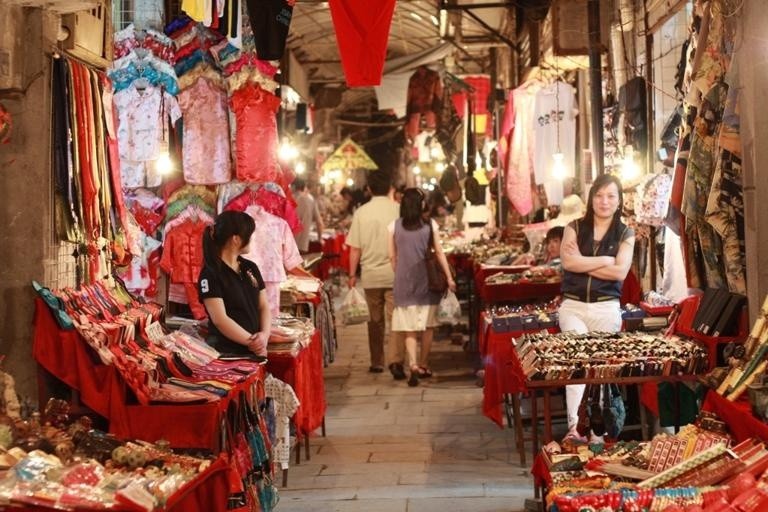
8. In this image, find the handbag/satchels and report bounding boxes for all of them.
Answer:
[604,396,626,437]
[576,402,591,437]
[221,450,249,511]
[425,251,448,292]
[257,416,275,479]
[243,426,280,512]
[227,432,262,512]
[592,399,606,437]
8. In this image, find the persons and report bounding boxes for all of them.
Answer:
[345,169,405,382]
[558,172,638,451]
[543,225,565,267]
[287,175,324,257]
[461,177,489,231]
[199,211,273,366]
[339,184,367,212]
[385,187,458,388]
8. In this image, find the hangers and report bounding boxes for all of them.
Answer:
[187,180,267,224]
[125,13,260,99]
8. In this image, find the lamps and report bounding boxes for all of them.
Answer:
[549,46,569,183]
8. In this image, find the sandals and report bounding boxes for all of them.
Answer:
[407,365,418,387]
[419,366,432,379]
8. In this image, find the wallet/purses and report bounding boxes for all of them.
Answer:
[514,330,708,381]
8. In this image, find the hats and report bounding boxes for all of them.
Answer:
[439,166,462,202]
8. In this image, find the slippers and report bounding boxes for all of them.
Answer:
[115,330,221,405]
[73,296,170,365]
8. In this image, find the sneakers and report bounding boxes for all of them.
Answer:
[369,366,383,372]
[150,355,268,404]
[32,276,145,329]
[389,363,406,380]
